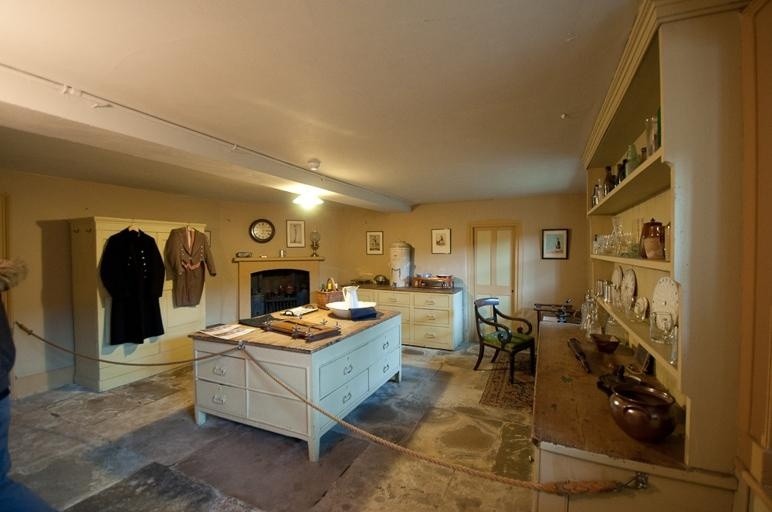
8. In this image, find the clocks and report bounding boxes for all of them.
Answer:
[249,219,275,243]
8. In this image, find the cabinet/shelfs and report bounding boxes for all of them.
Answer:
[64,215,208,394]
[412,287,463,352]
[341,285,380,312]
[529,438,735,512]
[380,286,412,346]
[588,0,757,467]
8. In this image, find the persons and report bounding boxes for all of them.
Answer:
[1,254,60,511]
[554,237,561,250]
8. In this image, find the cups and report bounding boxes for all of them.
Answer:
[651,311,675,345]
[594,231,637,258]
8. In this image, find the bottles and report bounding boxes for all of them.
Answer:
[578,287,602,340]
[591,143,641,210]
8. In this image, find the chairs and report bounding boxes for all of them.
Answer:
[473,298,535,383]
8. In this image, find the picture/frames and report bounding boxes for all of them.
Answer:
[430,228,452,254]
[285,220,307,248]
[542,229,568,260]
[365,230,384,255]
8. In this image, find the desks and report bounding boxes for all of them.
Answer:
[542,312,581,324]
[186,303,402,463]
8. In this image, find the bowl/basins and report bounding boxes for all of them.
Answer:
[324,301,377,319]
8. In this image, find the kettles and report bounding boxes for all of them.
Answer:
[326,278,337,292]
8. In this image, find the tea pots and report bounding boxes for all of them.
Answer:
[640,217,670,259]
[341,286,360,309]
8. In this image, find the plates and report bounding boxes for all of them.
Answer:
[651,276,679,332]
[611,265,623,289]
[620,269,637,310]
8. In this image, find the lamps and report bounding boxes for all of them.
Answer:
[291,189,325,213]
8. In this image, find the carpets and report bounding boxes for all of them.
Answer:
[478,346,538,415]
